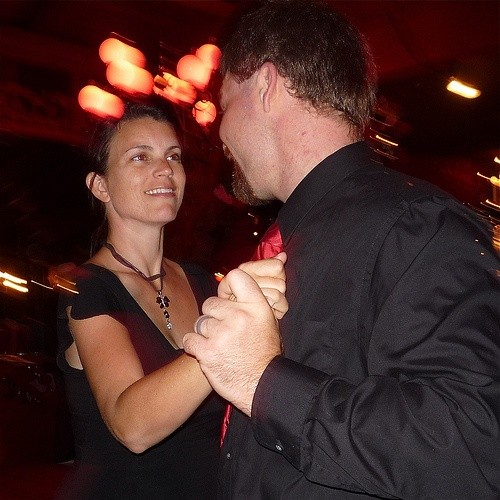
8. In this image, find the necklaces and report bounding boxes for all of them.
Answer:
[104,241,172,329]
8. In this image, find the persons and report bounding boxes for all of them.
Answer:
[216,0,500,500]
[56,96,289,500]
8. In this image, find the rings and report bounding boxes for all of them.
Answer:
[196,315,217,337]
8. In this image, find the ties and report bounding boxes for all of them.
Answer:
[219,216,283,448]
